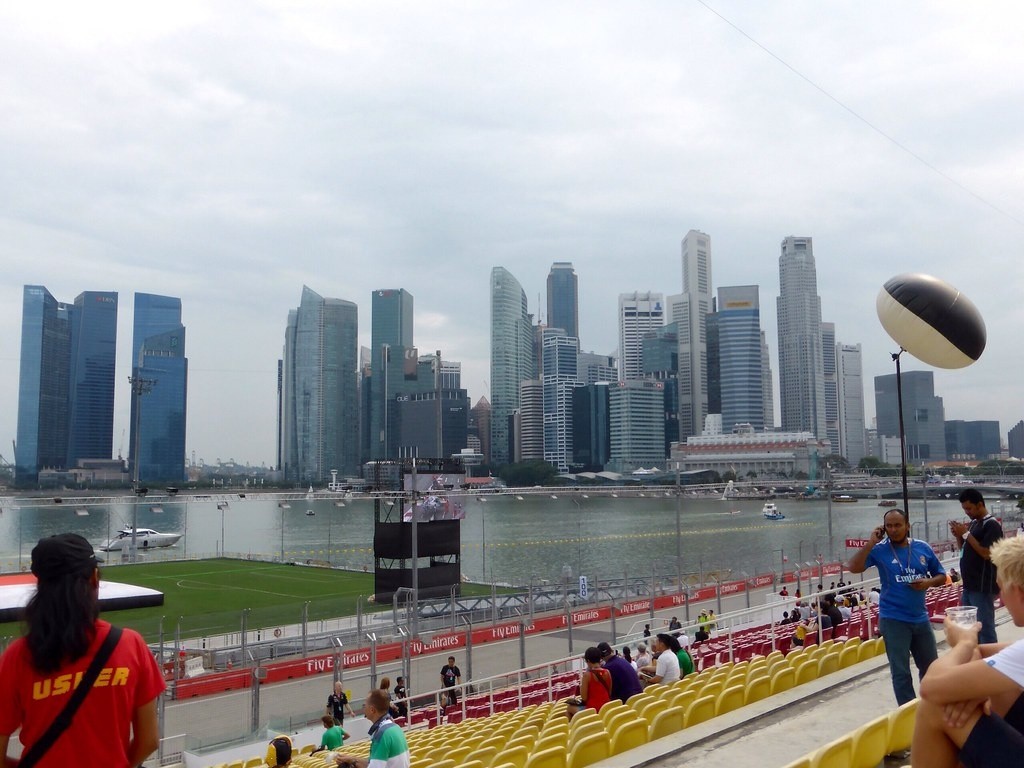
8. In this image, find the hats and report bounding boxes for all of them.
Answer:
[834,595,845,603]
[597,641,612,657]
[701,609,707,615]
[30,533,105,576]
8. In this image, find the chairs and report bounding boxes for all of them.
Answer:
[211,579,1005,768]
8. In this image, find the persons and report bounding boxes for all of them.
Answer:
[849,508,946,707]
[911,535,1024,768]
[596,642,643,705]
[707,610,716,629]
[616,632,694,690]
[394,677,408,717]
[440,656,461,704]
[380,677,400,719]
[326,682,355,728]
[567,647,612,723]
[669,617,681,638]
[950,568,960,583]
[694,627,709,644]
[697,609,710,633]
[779,578,881,649]
[336,689,410,768]
[644,624,651,645]
[320,716,351,751]
[948,488,1004,645]
[0,533,168,768]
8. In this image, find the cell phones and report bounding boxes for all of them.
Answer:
[877,528,886,537]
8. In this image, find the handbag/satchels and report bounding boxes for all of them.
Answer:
[564,697,586,706]
[440,683,448,708]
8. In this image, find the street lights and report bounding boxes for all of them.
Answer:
[127,373,159,549]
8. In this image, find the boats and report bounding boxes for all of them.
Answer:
[877,499,897,506]
[99,522,182,552]
[762,499,785,520]
[832,495,859,503]
[305,509,315,516]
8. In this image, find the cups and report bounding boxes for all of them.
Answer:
[945,605,979,627]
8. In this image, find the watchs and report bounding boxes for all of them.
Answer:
[962,531,970,540]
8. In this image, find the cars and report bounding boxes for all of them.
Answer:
[915,474,974,485]
[995,479,1024,484]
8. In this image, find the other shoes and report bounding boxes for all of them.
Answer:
[883,751,911,762]
